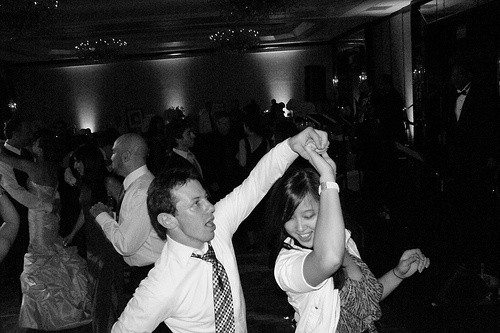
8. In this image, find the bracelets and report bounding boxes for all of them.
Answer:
[317,182,340,194]
[393,268,405,280]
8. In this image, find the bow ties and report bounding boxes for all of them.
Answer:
[456,80,475,97]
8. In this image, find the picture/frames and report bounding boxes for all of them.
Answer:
[127,109,143,130]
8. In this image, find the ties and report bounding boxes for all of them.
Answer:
[190,240,237,333]
[116,190,128,223]
[184,152,204,178]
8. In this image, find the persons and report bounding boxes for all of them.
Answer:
[110,126,330,333]
[0,188,21,262]
[0,142,94,330]
[89,133,167,333]
[0,50,500,255]
[62,143,124,333]
[274,137,431,333]
